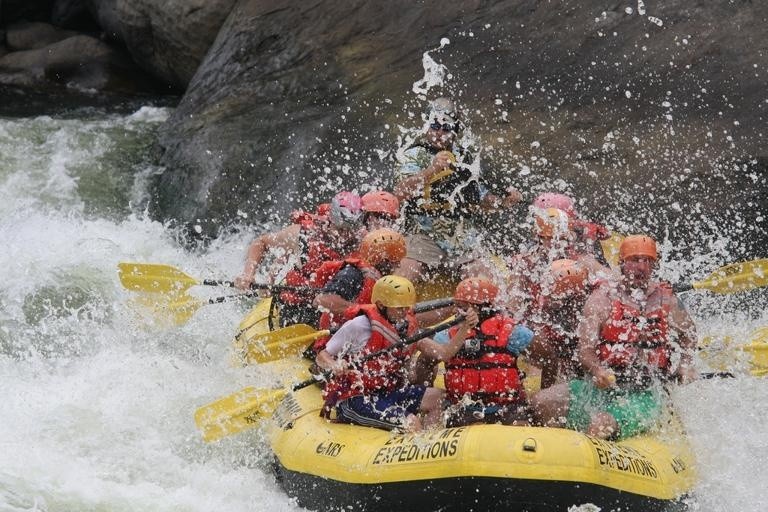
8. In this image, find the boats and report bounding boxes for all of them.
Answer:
[236,293,700,511]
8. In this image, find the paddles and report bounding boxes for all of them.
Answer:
[195,315,465,443]
[697,327,768,378]
[245,297,454,364]
[674,257,768,296]
[116,262,318,323]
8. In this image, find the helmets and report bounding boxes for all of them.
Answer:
[359,228,407,266]
[371,275,416,307]
[330,192,362,216]
[362,190,400,220]
[430,97,457,123]
[530,191,577,237]
[452,278,490,304]
[620,235,656,261]
[547,259,589,298]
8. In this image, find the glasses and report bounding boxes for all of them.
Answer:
[432,123,451,132]
